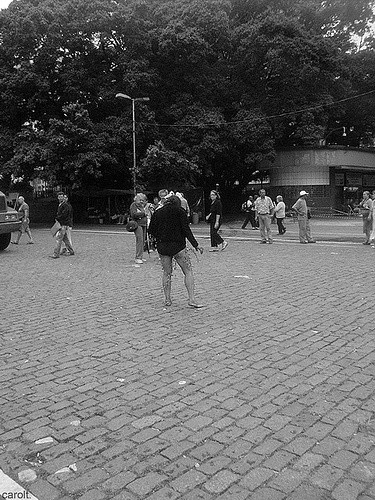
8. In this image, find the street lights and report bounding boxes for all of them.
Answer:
[116,93,150,197]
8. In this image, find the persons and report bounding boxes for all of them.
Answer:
[205,190,229,252]
[241,195,287,235]
[117,189,190,263]
[48,192,75,258]
[359,190,375,248]
[255,190,274,244]
[292,190,316,244]
[10,196,34,245]
[148,195,206,308]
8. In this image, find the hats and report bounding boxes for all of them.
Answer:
[299,191,309,196]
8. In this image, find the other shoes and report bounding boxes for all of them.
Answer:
[222,242,228,250]
[11,240,19,246]
[252,227,259,230]
[300,242,307,244]
[363,240,370,244]
[268,239,272,244]
[26,241,33,244]
[278,230,286,235]
[65,252,74,256]
[261,240,266,244]
[209,248,219,252]
[50,255,59,258]
[135,258,146,264]
[309,241,316,243]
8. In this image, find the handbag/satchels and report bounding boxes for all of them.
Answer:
[126,221,138,232]
[361,208,370,216]
